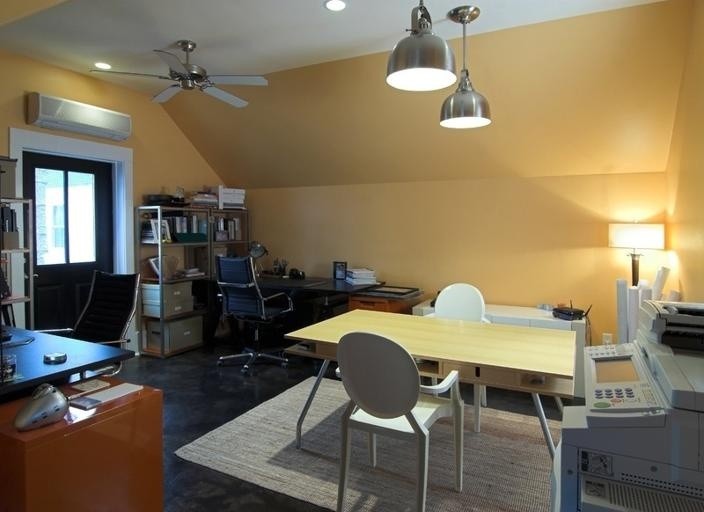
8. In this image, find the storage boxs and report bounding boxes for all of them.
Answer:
[142,296,194,318]
[147,316,203,353]
[141,281,192,301]
[0,158,17,199]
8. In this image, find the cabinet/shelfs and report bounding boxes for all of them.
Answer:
[412,299,587,412]
[349,285,421,313]
[0,199,34,329]
[135,206,211,359]
[211,209,249,337]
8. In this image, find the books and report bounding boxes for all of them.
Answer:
[61,379,111,401]
[141,185,246,278]
[345,267,376,285]
[69,382,144,410]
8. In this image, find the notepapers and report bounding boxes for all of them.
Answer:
[73,379,111,393]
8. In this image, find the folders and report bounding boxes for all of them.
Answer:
[2,207,12,231]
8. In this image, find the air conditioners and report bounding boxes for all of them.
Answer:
[26,91,132,141]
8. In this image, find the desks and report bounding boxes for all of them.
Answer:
[252,273,386,375]
[0,326,165,511]
[284,309,576,460]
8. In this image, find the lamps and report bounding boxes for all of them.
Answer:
[608,223,664,286]
[440,6,492,129]
[385,0,458,92]
[249,241,266,277]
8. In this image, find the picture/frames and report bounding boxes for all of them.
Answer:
[334,261,347,280]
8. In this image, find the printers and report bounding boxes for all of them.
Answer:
[559,299,703,511]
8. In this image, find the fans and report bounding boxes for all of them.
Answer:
[90,41,269,108]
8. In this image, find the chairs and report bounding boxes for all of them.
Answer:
[33,270,140,378]
[335,331,465,512]
[423,283,492,433]
[215,256,294,374]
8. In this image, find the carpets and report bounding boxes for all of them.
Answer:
[174,376,563,512]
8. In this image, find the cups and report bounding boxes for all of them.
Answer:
[0,355,16,382]
